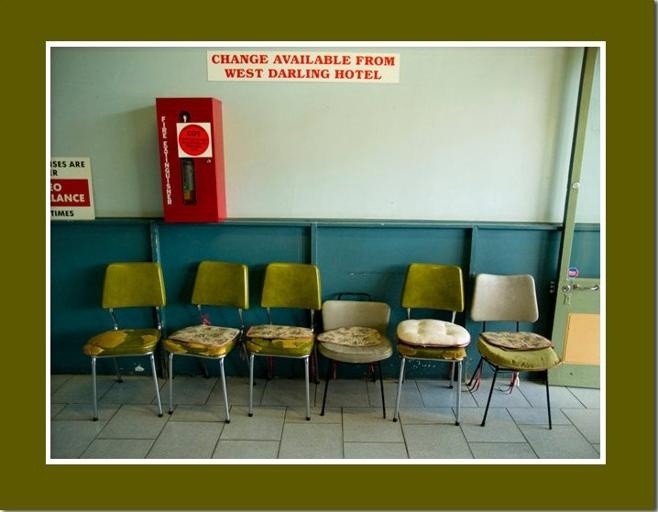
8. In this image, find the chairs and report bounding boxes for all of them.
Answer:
[82,260,562,430]
